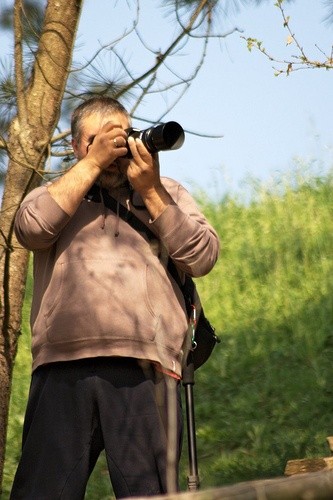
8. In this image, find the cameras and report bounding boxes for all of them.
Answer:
[116,120,185,159]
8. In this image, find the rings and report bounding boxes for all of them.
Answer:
[113,138,117,147]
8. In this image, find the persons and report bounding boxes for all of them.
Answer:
[9,97,220,500]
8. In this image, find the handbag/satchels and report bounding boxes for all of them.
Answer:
[184,274,218,371]
